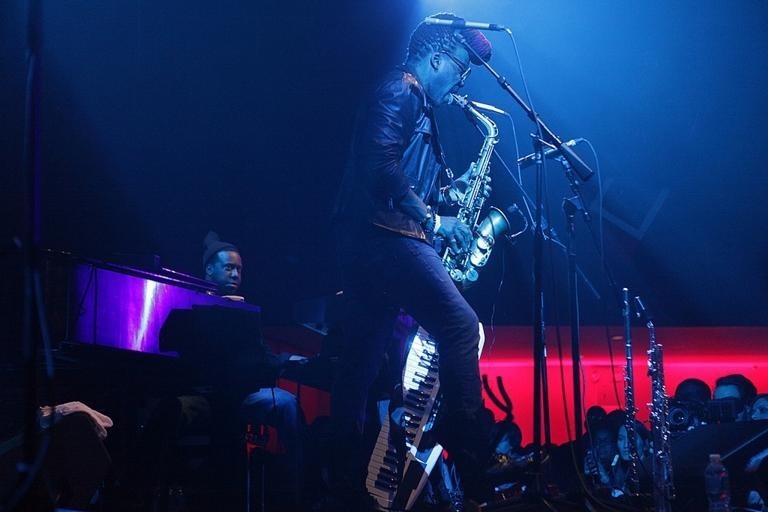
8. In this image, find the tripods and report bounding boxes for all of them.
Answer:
[456,34,594,512]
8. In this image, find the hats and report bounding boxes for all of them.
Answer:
[203,242,235,266]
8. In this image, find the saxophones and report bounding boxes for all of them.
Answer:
[622,288,639,511]
[635,294,678,511]
[441,92,511,292]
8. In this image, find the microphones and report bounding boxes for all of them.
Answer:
[424,17,499,31]
[516,139,576,169]
[470,101,505,114]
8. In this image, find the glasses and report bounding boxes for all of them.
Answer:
[441,49,472,81]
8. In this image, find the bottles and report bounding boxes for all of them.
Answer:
[703,455,730,508]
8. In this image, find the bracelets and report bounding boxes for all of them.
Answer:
[441,184,454,208]
[419,206,441,234]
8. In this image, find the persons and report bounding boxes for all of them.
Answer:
[450,374,767,510]
[328,13,492,510]
[200,241,308,493]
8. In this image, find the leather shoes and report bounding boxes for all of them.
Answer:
[462,449,549,503]
